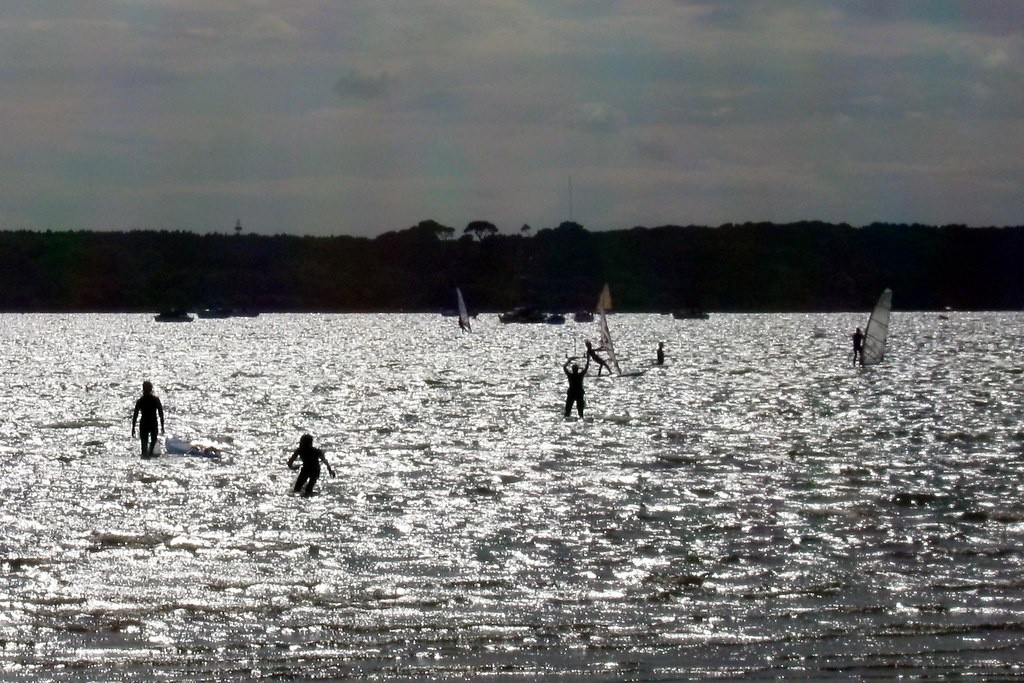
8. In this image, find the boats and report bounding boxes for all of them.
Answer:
[154,306,194,322]
[198,308,230,318]
[575,311,594,321]
[671,307,709,319]
[543,314,566,324]
[498,308,546,323]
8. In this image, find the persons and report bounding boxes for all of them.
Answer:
[132,381,165,455]
[288,434,335,497]
[657,342,664,364]
[563,357,588,418]
[853,327,866,366]
[585,341,612,376]
